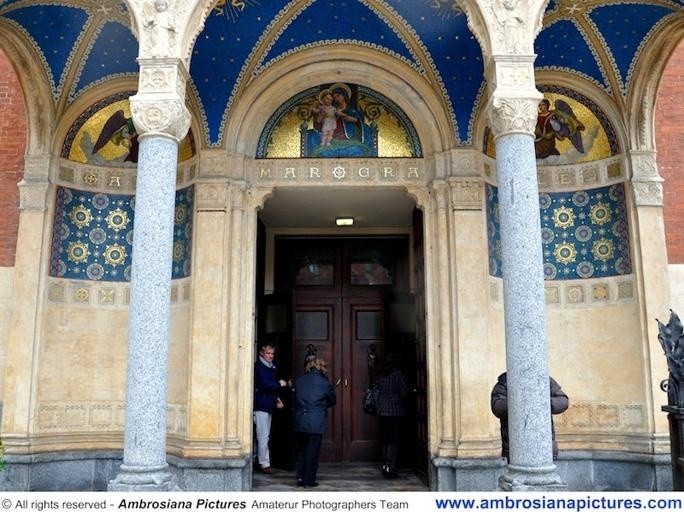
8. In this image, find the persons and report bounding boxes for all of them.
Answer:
[534,99,571,159]
[363,351,409,481]
[491,372,568,465]
[308,83,363,148]
[291,343,337,488]
[123,118,138,164]
[253,344,287,474]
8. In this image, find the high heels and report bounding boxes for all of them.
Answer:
[382,464,402,480]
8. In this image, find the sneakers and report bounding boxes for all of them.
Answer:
[260,468,273,474]
[297,477,318,488]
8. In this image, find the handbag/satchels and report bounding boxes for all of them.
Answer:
[362,383,380,416]
